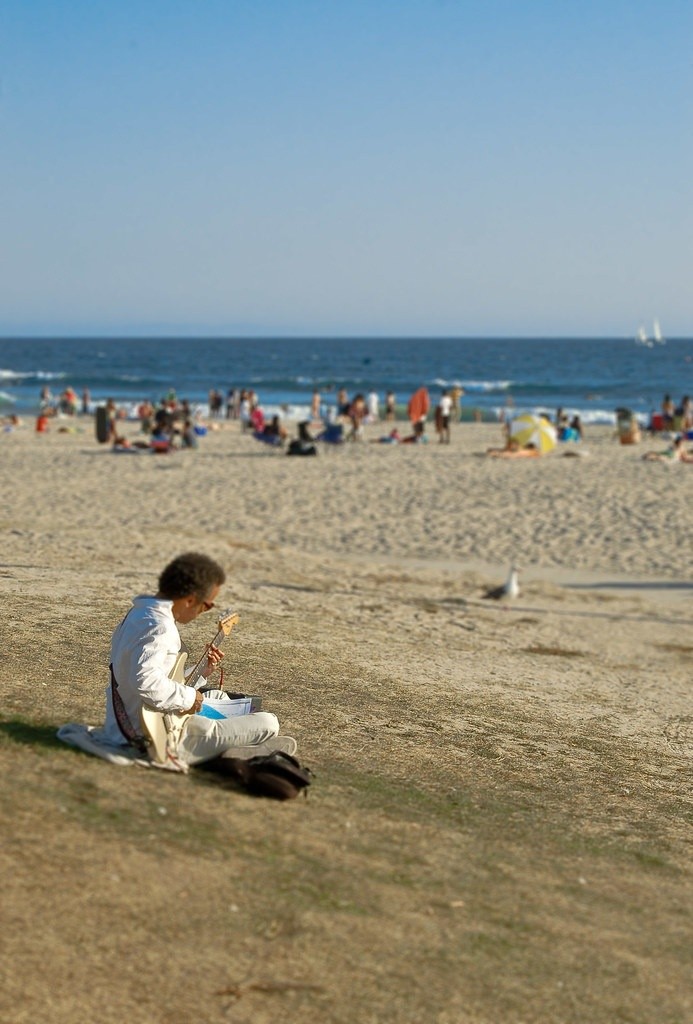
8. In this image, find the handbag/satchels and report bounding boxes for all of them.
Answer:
[236,749,316,801]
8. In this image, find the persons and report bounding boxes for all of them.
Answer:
[0,385,693,465]
[436,389,452,443]
[109,553,296,772]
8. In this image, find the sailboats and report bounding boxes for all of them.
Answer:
[633,325,653,349]
[651,318,666,345]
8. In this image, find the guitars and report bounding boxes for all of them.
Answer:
[138,607,241,764]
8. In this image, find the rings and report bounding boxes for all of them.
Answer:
[211,663,215,666]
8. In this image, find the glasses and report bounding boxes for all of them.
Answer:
[195,595,214,612]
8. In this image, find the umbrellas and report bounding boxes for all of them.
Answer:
[510,411,557,455]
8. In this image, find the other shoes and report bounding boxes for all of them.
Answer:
[221,735,297,763]
